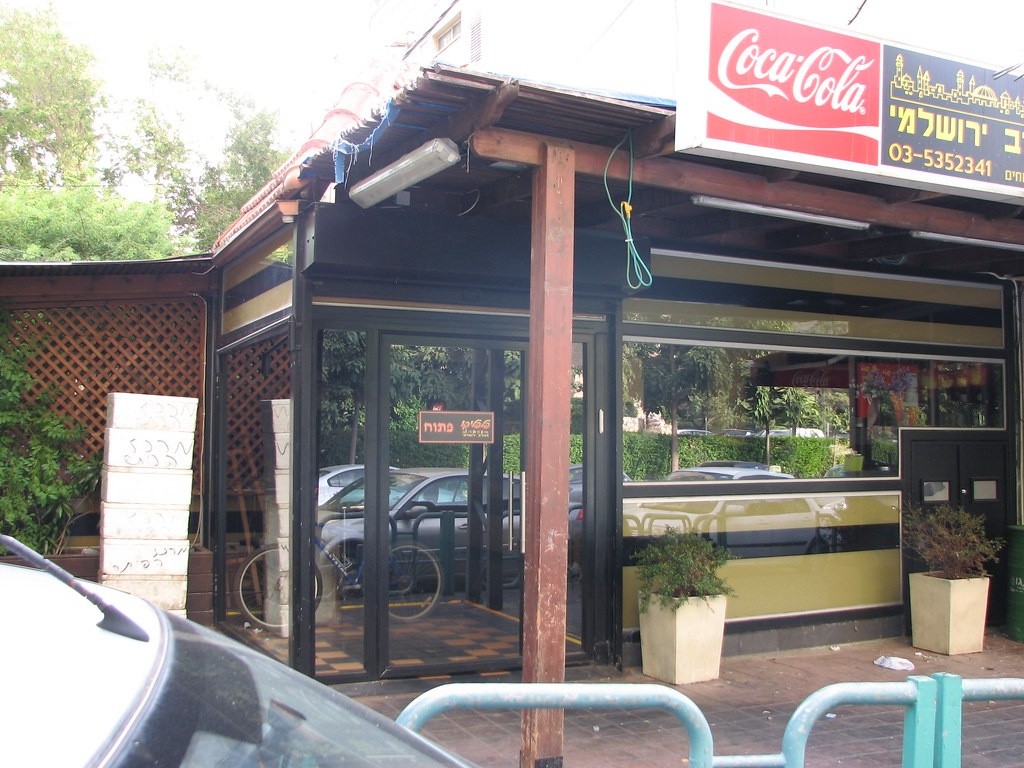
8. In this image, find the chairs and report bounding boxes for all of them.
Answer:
[424,484,439,504]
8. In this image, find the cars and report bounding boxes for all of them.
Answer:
[674,429,714,438]
[700,461,770,471]
[821,461,893,479]
[1,530,482,768]
[663,468,796,484]
[315,461,397,510]
[717,428,849,439]
[569,463,633,502]
[316,466,582,580]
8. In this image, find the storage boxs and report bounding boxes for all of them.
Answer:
[99,393,200,619]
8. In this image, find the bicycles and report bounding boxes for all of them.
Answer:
[232,514,443,631]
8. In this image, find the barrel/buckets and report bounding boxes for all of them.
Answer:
[1005,526,1024,642]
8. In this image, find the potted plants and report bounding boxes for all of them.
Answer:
[896,493,1005,655]
[0,322,216,628]
[627,524,736,684]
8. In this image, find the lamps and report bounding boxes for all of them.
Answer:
[349,138,462,209]
